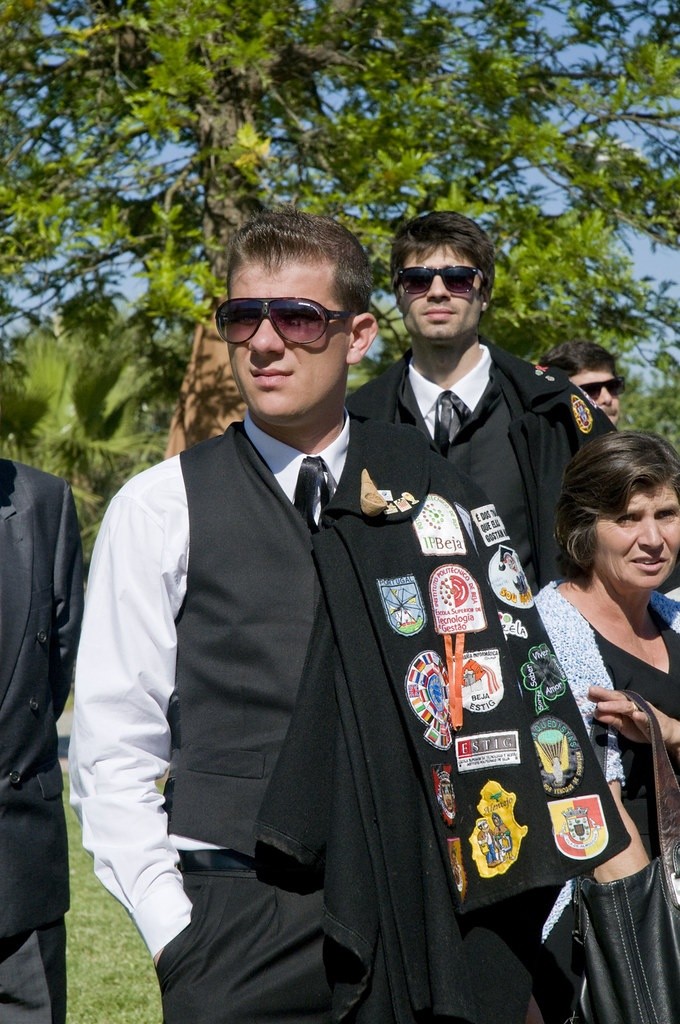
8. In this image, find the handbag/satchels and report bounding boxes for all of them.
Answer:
[571,690,680,1024]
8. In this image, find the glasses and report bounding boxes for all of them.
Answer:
[395,265,484,295]
[215,295,356,345]
[578,377,624,399]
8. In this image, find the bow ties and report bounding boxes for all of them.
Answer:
[293,456,337,536]
[433,391,473,458]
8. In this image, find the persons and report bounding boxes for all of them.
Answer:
[66,216,631,1024]
[346,211,620,587]
[539,341,620,427]
[531,430,680,990]
[0,461,86,1024]
